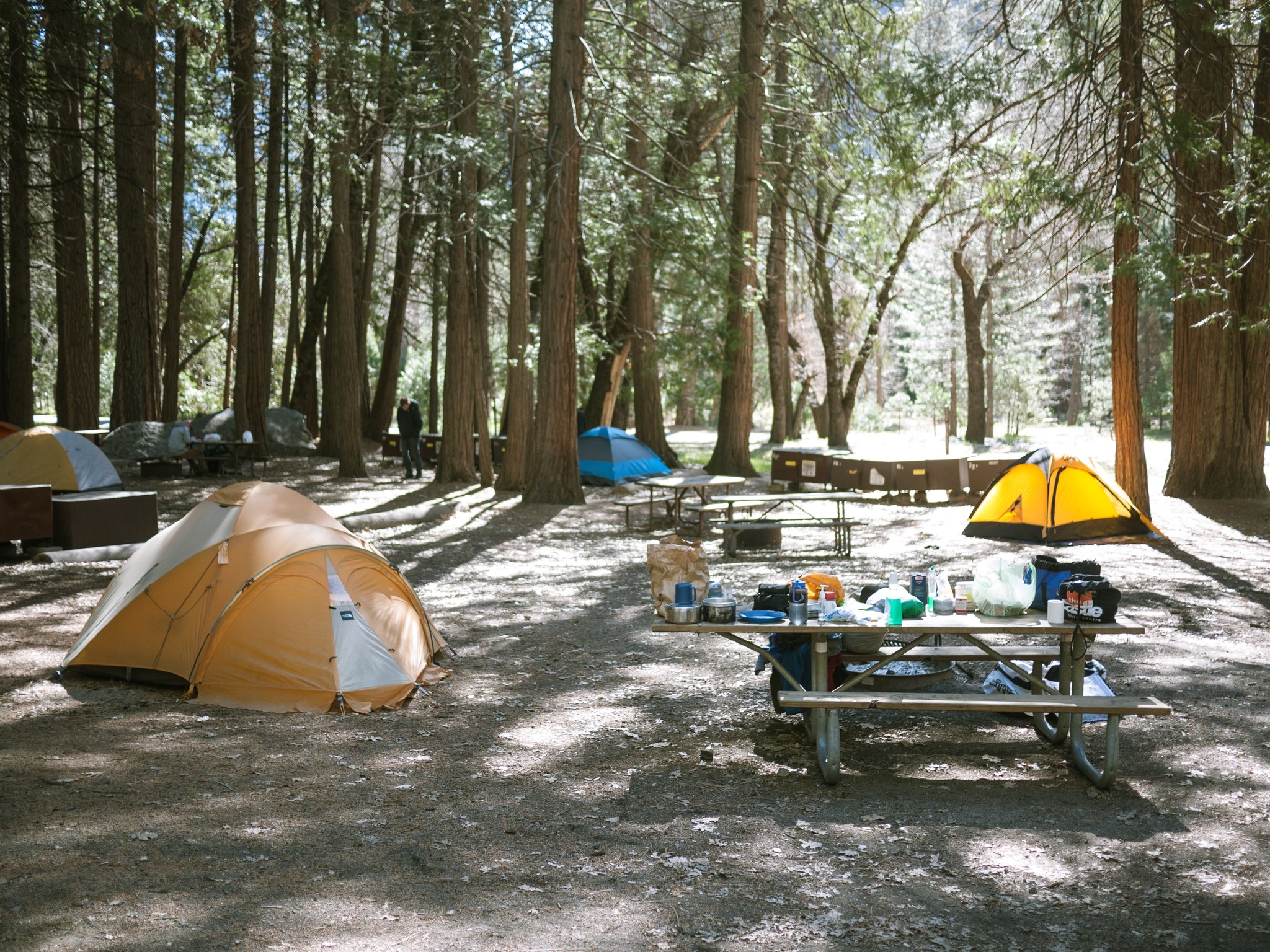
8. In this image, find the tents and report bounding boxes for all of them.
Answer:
[59,480,452,713]
[577,427,671,488]
[0,425,122,492]
[961,447,1170,546]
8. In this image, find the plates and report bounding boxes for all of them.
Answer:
[787,606,819,618]
[737,610,786,622]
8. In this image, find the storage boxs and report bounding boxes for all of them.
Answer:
[382,432,507,465]
[772,449,1027,491]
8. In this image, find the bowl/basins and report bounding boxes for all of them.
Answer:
[807,599,819,611]
[703,597,736,623]
[664,601,702,624]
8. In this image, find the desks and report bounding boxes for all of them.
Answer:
[626,474,745,537]
[711,491,862,556]
[652,607,1144,789]
[183,440,267,477]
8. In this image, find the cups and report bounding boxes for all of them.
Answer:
[825,599,837,616]
[675,583,697,604]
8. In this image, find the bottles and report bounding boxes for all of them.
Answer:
[789,580,808,624]
[927,566,936,611]
[954,581,974,600]
[885,572,903,625]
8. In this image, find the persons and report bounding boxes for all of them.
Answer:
[396,397,424,479]
[167,421,210,476]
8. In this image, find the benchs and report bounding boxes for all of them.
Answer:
[614,493,868,557]
[760,645,1171,787]
[172,454,273,477]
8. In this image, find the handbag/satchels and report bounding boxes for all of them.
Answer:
[981,658,1123,722]
[971,554,1037,617]
[1055,574,1122,624]
[787,573,845,606]
[865,583,925,617]
[753,583,791,615]
[1031,555,1101,610]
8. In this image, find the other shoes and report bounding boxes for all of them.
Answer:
[415,472,422,479]
[403,474,413,478]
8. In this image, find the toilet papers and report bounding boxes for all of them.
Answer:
[1047,599,1065,623]
